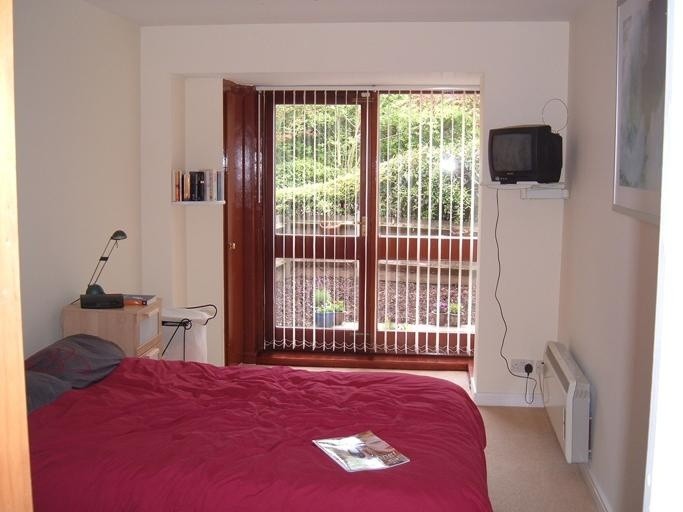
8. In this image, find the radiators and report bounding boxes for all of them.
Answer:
[542,340,590,464]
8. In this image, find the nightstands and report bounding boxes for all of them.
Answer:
[61,294,162,358]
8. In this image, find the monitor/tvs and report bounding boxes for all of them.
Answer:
[488,124,563,184]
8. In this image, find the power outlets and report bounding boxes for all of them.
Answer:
[509,359,536,375]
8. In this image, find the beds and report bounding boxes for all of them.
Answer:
[24,356,491,512]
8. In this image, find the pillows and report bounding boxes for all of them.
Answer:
[25,335,125,417]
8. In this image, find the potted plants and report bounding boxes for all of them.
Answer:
[312,289,344,327]
[448,304,459,326]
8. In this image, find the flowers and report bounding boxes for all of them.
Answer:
[434,301,446,312]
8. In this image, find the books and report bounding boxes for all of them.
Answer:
[171,168,224,202]
[122,293,158,306]
[310,429,411,473]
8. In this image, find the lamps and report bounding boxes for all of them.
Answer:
[79,229,127,308]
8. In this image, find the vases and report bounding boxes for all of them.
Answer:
[432,309,446,325]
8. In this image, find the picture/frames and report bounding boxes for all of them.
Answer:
[610,2,661,224]
